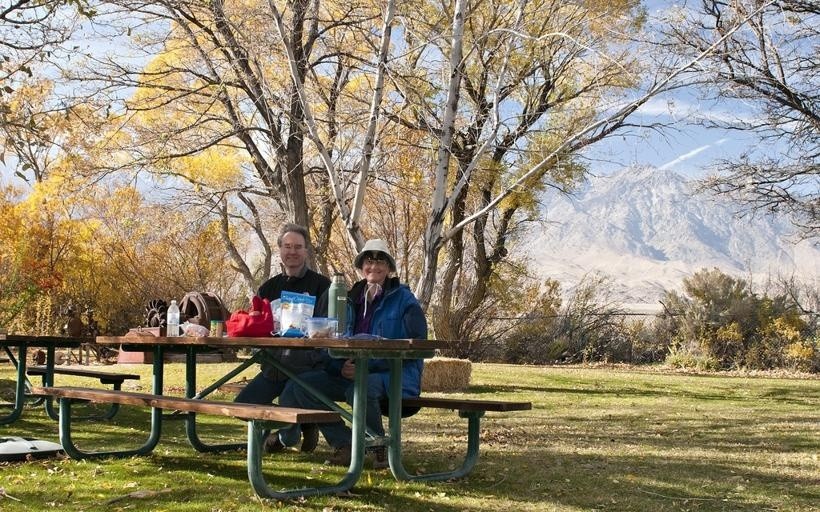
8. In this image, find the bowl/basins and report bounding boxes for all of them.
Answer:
[305,316,339,339]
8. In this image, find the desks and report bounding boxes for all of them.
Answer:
[0,335,86,421]
[95,334,452,497]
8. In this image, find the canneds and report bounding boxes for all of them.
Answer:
[210,320,224,337]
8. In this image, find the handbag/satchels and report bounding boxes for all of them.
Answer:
[226,296,273,337]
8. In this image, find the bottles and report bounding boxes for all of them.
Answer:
[166,299,180,337]
[327,271,349,339]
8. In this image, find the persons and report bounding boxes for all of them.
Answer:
[294,238,427,470]
[232,223,333,452]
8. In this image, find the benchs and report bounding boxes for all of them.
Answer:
[28,363,140,421]
[220,378,532,481]
[27,385,353,500]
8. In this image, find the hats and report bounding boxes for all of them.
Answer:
[354,240,396,272]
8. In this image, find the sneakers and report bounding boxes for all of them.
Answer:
[264,431,284,453]
[301,423,318,452]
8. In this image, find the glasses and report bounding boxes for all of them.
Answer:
[365,258,386,265]
